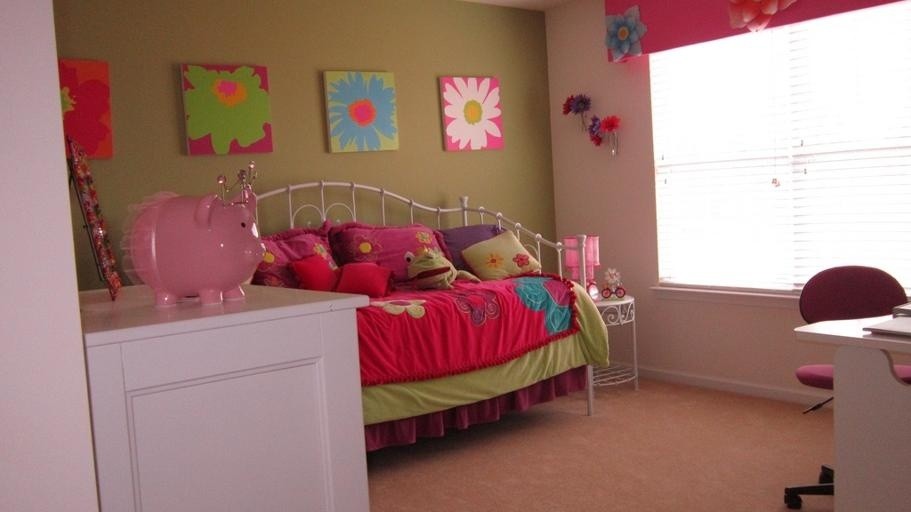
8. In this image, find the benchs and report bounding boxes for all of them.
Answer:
[255,180,593,451]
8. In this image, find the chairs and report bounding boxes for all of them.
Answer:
[783,266,911,510]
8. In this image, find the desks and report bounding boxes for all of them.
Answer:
[793,315,911,512]
[587,294,639,391]
[79,285,370,512]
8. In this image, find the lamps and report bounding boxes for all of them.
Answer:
[562,235,600,284]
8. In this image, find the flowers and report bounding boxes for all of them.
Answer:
[562,93,622,159]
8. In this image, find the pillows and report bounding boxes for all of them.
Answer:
[257,219,542,298]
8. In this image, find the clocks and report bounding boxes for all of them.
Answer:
[587,283,600,302]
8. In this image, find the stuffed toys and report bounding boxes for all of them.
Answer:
[407,248,482,291]
